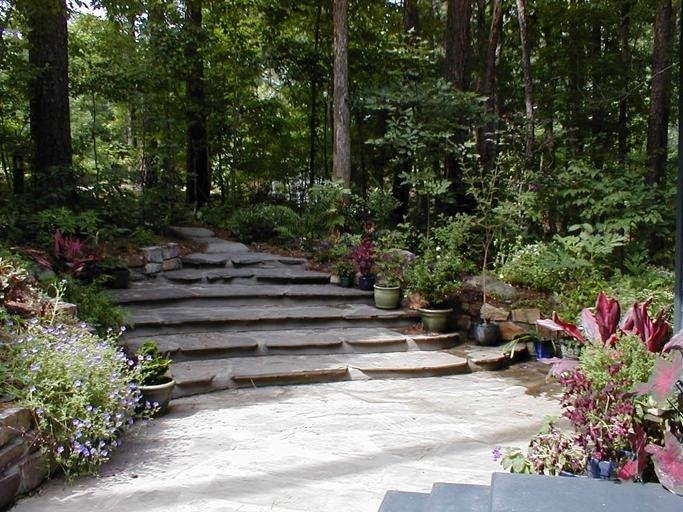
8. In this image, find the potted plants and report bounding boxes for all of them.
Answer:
[335,233,462,331]
[100,252,129,289]
[448,139,532,346]
[124,340,176,419]
